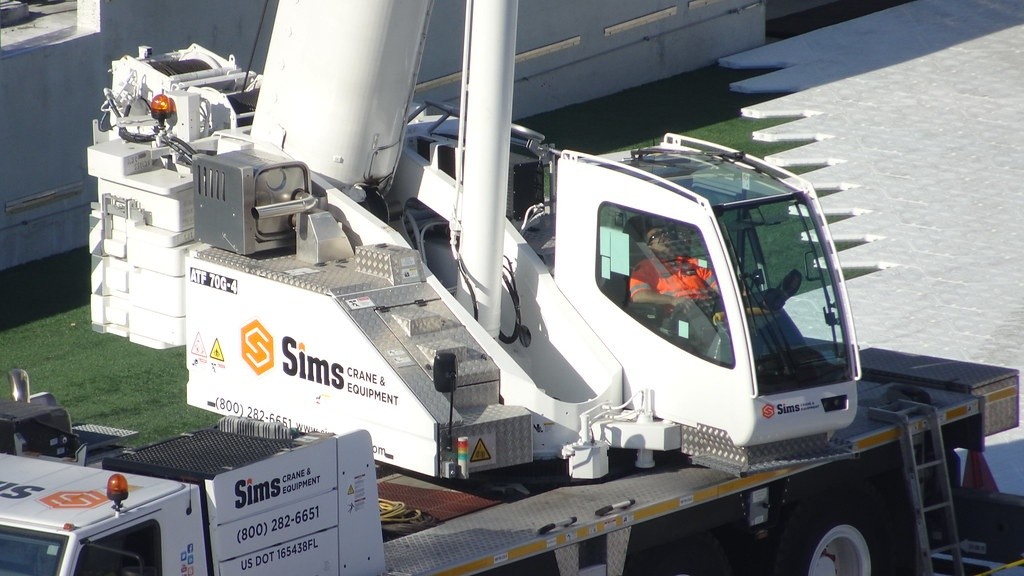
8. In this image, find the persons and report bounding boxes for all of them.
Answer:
[630,226,802,354]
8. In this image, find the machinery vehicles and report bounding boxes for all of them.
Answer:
[80,0,868,484]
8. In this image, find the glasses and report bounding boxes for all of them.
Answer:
[647,229,668,246]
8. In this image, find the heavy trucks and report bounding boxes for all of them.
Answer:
[0,337,1021,576]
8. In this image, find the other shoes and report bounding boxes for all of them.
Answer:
[770,269,803,309]
